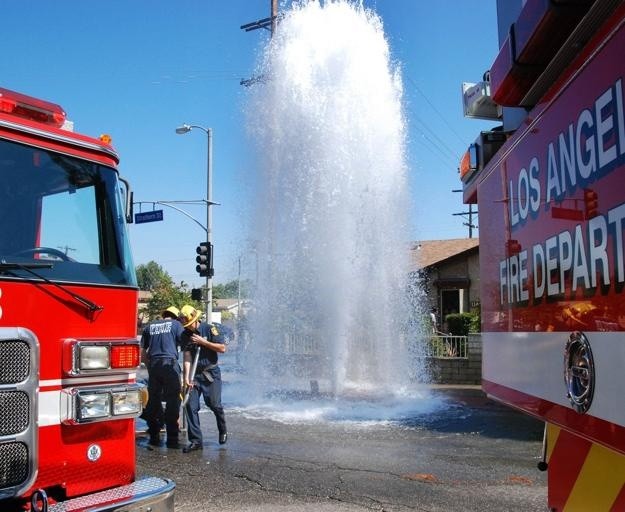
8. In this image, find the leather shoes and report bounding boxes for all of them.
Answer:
[219,433,227,444]
[183,442,203,453]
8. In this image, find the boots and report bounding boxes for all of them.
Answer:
[148,429,163,446]
[167,433,182,448]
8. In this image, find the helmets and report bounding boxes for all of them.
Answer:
[179,305,201,327]
[163,306,180,318]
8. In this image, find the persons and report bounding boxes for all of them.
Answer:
[430,305,443,336]
[136,305,190,448]
[177,304,229,453]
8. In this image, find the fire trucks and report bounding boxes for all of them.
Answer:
[0,82,179,511]
[455,1,625,512]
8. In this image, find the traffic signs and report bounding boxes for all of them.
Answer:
[134,209,164,225]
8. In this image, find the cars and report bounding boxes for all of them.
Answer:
[210,321,235,345]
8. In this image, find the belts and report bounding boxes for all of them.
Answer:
[196,360,218,383]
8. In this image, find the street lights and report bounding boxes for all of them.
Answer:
[172,120,214,328]
[237,248,258,320]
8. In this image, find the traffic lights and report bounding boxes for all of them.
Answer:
[194,242,213,277]
[191,287,204,301]
